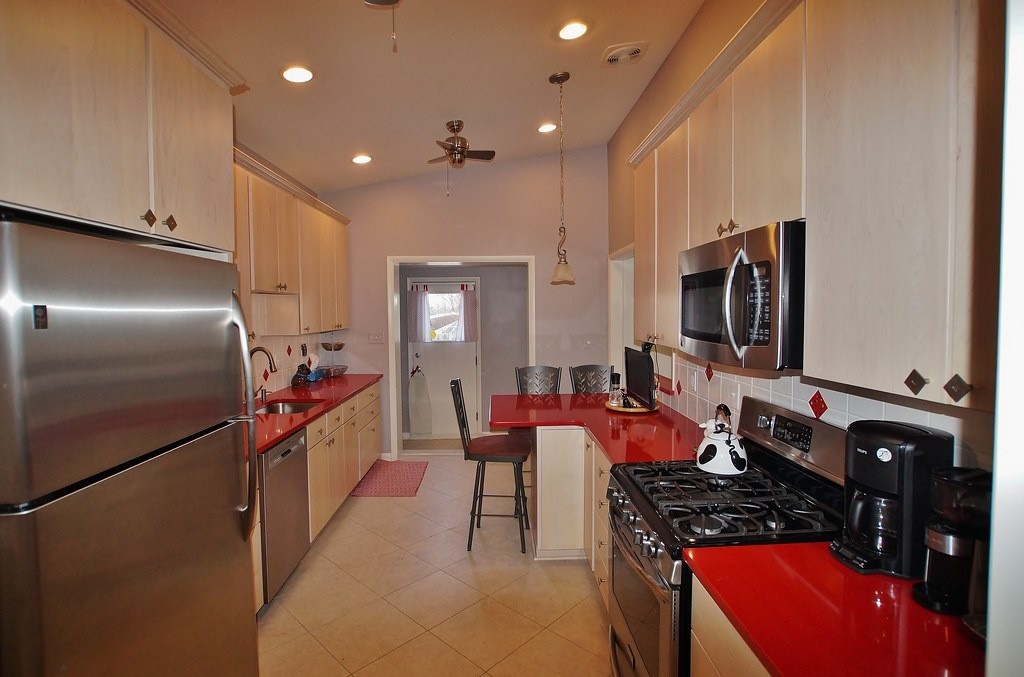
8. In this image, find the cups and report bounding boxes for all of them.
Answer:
[609,390,621,406]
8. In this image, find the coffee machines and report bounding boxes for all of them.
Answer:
[828,419,954,579]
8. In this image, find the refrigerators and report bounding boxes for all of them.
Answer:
[0,220,258,677]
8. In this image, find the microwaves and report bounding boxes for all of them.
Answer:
[678,221,805,371]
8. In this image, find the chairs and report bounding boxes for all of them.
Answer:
[569,364,615,394]
[514,366,562,394]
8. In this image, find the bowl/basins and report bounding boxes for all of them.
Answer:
[317,365,347,376]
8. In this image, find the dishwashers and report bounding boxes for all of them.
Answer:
[257,427,310,603]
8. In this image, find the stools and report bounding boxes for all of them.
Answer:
[450,379,533,553]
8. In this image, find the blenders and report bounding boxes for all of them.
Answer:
[912,466,991,615]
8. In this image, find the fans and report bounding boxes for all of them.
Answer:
[429,121,497,164]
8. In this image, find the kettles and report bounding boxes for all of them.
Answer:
[696,403,747,475]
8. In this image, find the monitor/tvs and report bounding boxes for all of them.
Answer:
[624,347,656,412]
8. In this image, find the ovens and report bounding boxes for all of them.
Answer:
[608,505,691,677]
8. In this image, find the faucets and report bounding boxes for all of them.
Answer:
[261,389,273,403]
[244,346,277,409]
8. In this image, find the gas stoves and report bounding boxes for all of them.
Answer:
[610,394,846,558]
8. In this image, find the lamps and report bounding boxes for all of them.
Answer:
[551,71,574,285]
[447,153,467,168]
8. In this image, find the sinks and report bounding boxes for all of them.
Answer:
[254,402,321,414]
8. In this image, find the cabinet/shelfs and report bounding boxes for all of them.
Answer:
[251,170,299,294]
[0,0,238,255]
[800,0,1006,413]
[322,213,347,331]
[299,200,321,334]
[583,433,609,607]
[308,381,383,542]
[690,0,806,250]
[237,164,249,336]
[632,118,693,349]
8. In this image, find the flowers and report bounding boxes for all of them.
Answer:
[690,370,696,391]
[730,381,740,411]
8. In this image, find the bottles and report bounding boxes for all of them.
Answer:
[611,373,620,390]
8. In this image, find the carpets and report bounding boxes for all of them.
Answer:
[351,458,429,497]
